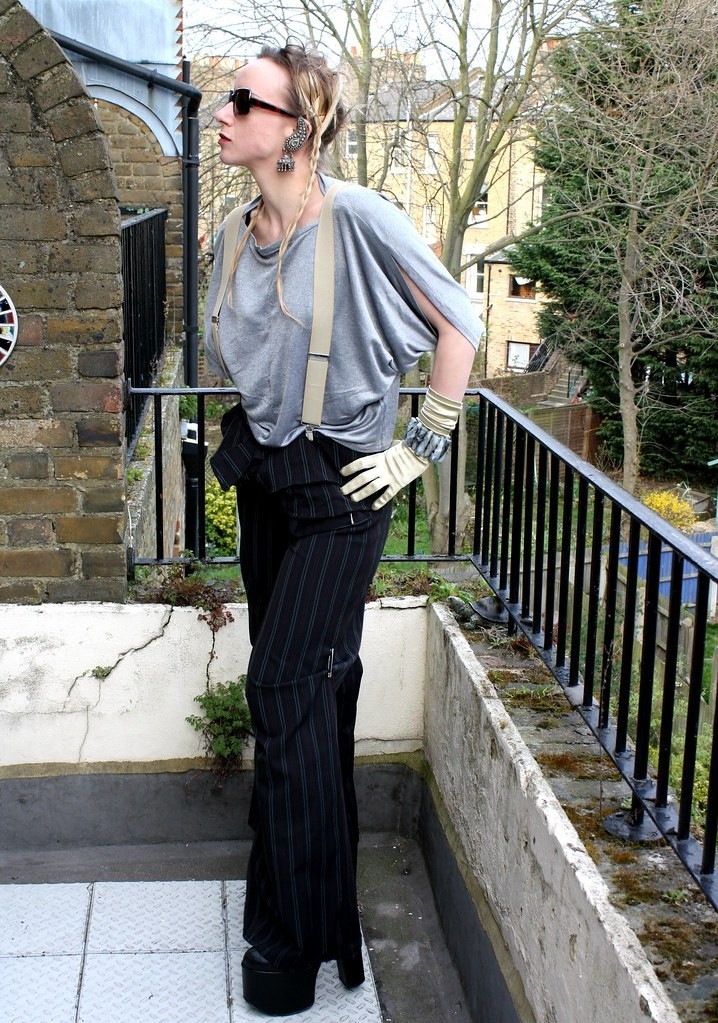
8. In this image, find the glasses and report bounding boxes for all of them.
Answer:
[228,88,315,139]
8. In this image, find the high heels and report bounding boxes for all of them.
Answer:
[241,930,366,1017]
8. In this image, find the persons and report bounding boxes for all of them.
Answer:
[203,43,481,1013]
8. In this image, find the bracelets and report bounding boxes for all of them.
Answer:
[405,416,451,464]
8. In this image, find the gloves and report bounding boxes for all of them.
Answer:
[339,384,463,510]
